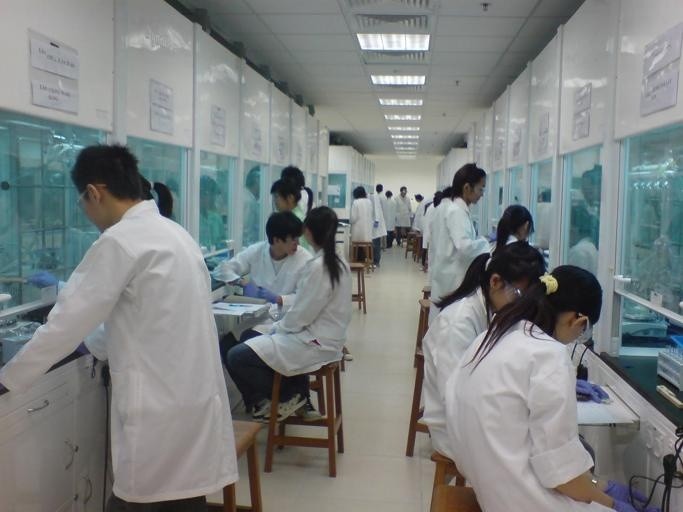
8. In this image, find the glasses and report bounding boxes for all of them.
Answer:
[501,281,525,304]
[75,183,109,206]
[577,312,594,342]
[402,190,407,193]
[470,184,487,196]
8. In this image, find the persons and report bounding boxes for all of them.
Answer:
[244,166,259,244]
[427,164,497,326]
[580,165,601,206]
[487,206,534,269]
[0,143,239,512]
[568,207,599,274]
[26,174,172,362]
[0,175,86,267]
[270,166,433,270]
[422,241,609,473]
[212,210,322,419]
[198,175,228,250]
[446,265,659,512]
[226,206,352,424]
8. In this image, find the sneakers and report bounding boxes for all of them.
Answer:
[343,347,354,362]
[251,391,323,425]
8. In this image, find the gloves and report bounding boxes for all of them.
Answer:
[27,270,60,294]
[485,229,499,243]
[576,379,610,404]
[602,479,661,512]
[373,221,378,229]
[242,282,278,305]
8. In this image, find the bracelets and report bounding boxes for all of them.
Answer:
[236,277,243,285]
[592,475,598,489]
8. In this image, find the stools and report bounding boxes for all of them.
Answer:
[348,260,368,313]
[430,453,466,486]
[400,346,431,457]
[415,298,435,365]
[426,484,482,512]
[255,355,350,476]
[204,415,264,510]
[419,284,431,299]
[352,227,425,272]
